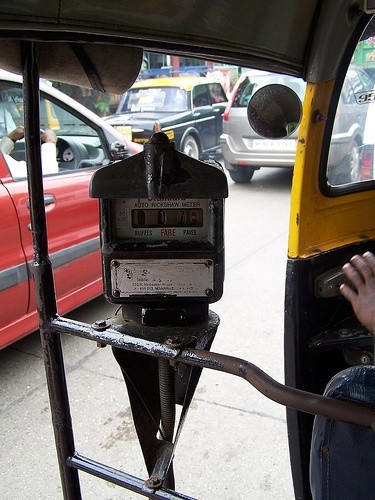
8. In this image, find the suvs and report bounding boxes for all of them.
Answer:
[98,66,228,162]
[218,64,375,185]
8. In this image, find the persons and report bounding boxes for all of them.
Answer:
[340,251,375,334]
[0,125,59,178]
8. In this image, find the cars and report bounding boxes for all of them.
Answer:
[0,66,143,354]
